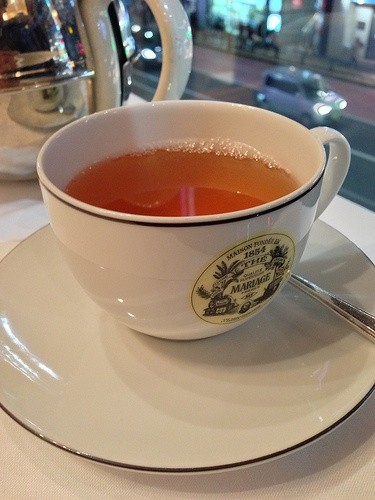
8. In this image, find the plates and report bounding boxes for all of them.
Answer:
[0,222,375,476]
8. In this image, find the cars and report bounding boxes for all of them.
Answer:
[124,22,164,73]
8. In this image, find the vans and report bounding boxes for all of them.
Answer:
[255,66,347,130]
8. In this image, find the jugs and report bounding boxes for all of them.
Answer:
[0,0,194,183]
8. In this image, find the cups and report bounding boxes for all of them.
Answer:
[36,99,351,341]
